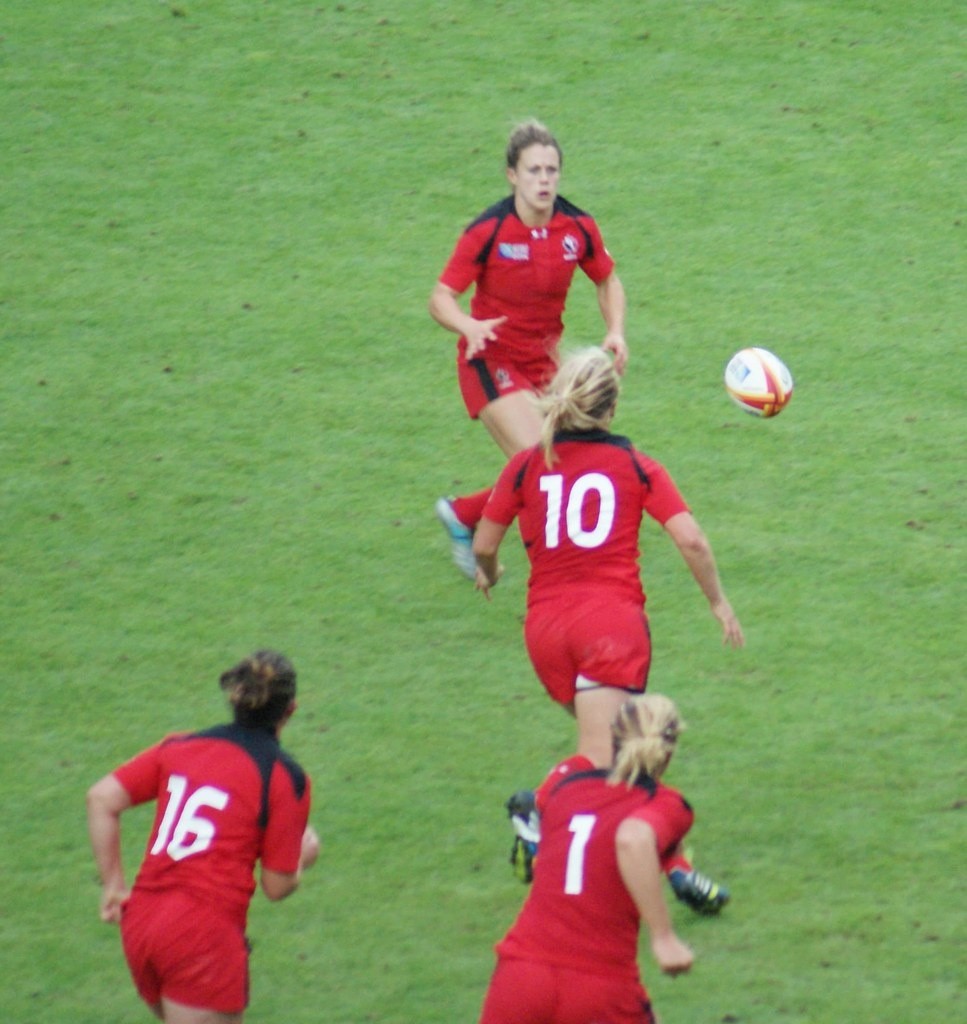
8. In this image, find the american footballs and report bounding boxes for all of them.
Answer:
[726,347,794,419]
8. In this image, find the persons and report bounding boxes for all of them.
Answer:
[428,121,630,580]
[469,348,747,1024]
[84,648,322,1024]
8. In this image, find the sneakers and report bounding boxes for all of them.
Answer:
[667,866,731,917]
[433,493,477,583]
[503,787,543,886]
